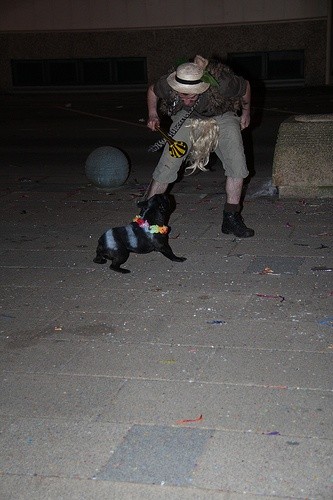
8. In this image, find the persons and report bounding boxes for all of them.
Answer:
[145,55,256,239]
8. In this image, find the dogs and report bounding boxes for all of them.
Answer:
[93,193,187,274]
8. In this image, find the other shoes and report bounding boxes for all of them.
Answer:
[222,211,254,237]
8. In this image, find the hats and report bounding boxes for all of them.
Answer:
[166,62,210,93]
